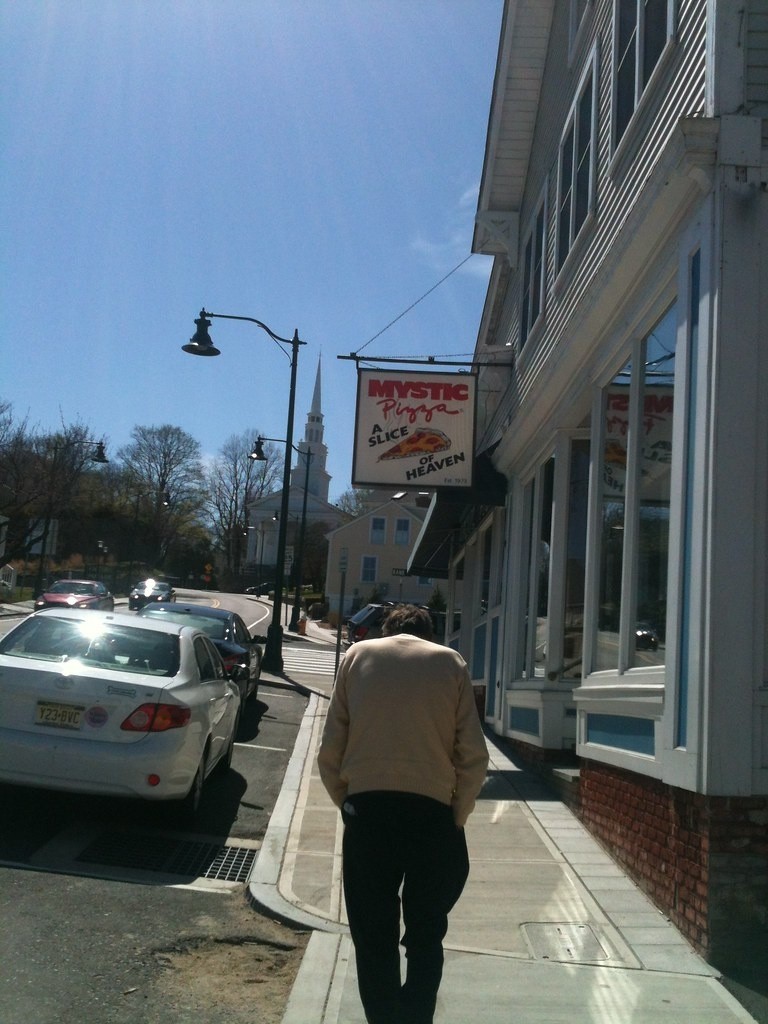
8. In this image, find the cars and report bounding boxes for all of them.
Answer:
[244,582,277,596]
[0,604,253,819]
[32,579,116,615]
[99,600,269,725]
[128,578,177,611]
[637,626,659,653]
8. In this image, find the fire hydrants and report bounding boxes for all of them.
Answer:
[296,613,308,635]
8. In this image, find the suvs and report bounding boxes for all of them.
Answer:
[342,602,461,651]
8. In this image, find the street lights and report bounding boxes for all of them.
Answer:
[97,539,104,581]
[248,526,264,598]
[246,433,316,632]
[123,489,172,597]
[102,546,109,582]
[182,306,311,675]
[32,440,110,600]
[272,508,301,592]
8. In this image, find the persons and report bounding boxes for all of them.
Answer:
[318,605,489,1024]
